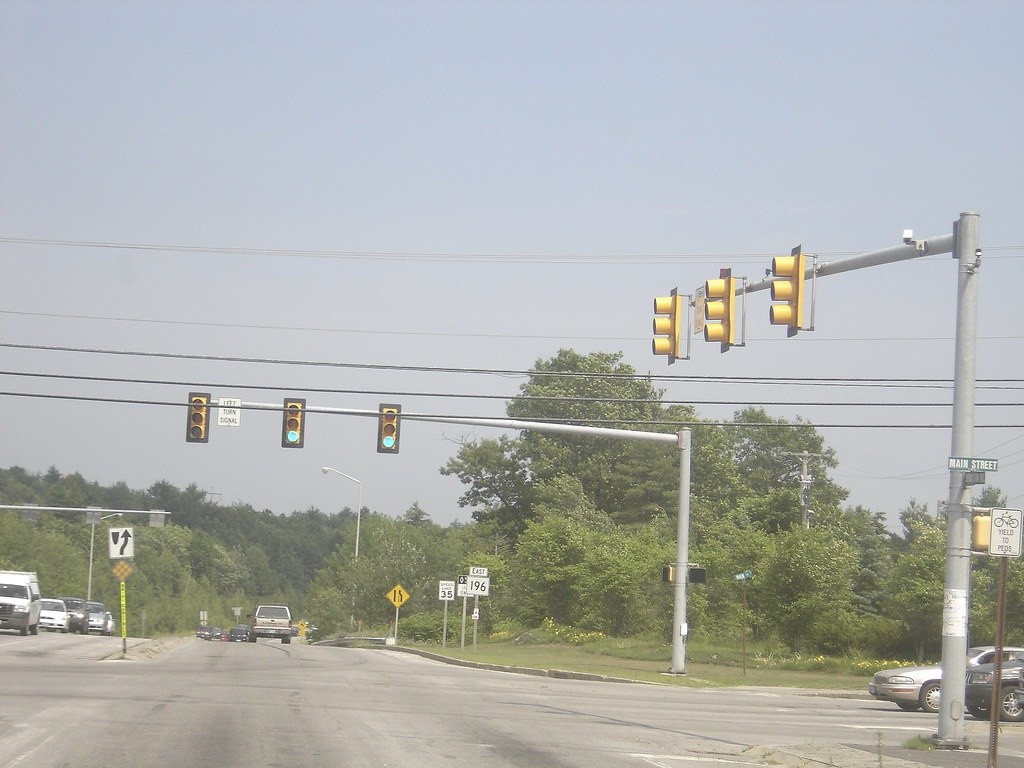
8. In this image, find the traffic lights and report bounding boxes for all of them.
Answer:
[770,244,806,339]
[704,267,734,353]
[376,403,402,454]
[282,397,306,449]
[652,286,682,365]
[186,392,211,443]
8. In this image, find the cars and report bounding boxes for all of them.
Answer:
[107,615,117,636]
[963,652,1024,723]
[195,626,249,642]
[866,645,1024,713]
[38,597,71,635]
[85,601,111,636]
[57,595,90,635]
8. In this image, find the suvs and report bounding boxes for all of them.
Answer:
[245,604,293,644]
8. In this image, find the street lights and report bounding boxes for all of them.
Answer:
[322,466,363,632]
[86,512,124,602]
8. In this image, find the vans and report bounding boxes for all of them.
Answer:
[0,569,43,637]
